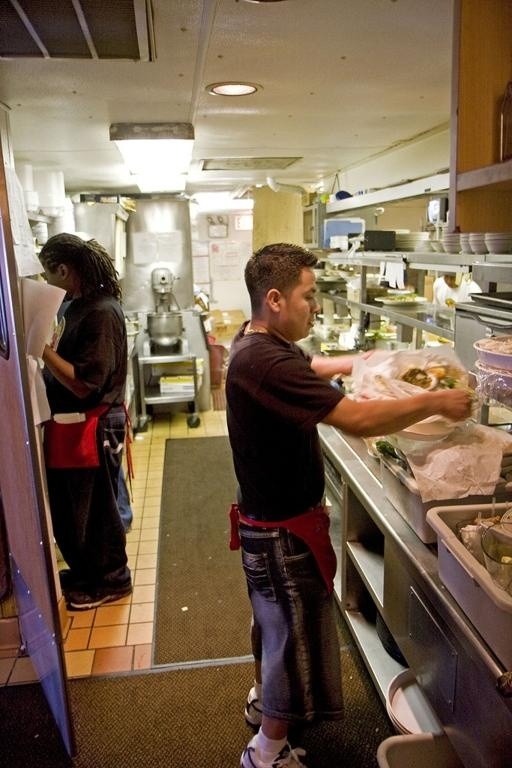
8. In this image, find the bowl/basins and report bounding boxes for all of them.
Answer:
[429,233,511,255]
[312,269,327,281]
[338,270,355,278]
[473,335,512,399]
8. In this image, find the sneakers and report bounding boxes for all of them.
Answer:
[240,735,308,767]
[244,687,266,725]
[59,570,133,610]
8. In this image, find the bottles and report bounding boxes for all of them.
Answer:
[496,82,511,164]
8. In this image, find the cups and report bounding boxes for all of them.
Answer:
[456,518,512,597]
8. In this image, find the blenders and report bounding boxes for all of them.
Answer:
[147,267,185,349]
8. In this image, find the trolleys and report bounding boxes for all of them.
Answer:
[137,336,201,431]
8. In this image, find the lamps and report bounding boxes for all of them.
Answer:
[109,122,196,194]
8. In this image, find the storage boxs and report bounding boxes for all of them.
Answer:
[372,442,512,544]
[426,501,512,673]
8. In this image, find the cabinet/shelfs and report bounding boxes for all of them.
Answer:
[316,251,486,350]
[301,204,325,250]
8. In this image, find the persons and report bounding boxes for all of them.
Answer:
[224,244,474,768]
[35,230,134,612]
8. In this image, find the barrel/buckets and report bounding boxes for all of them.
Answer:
[147,312,184,347]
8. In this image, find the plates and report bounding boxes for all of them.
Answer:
[321,274,340,280]
[374,294,427,307]
[395,231,427,250]
